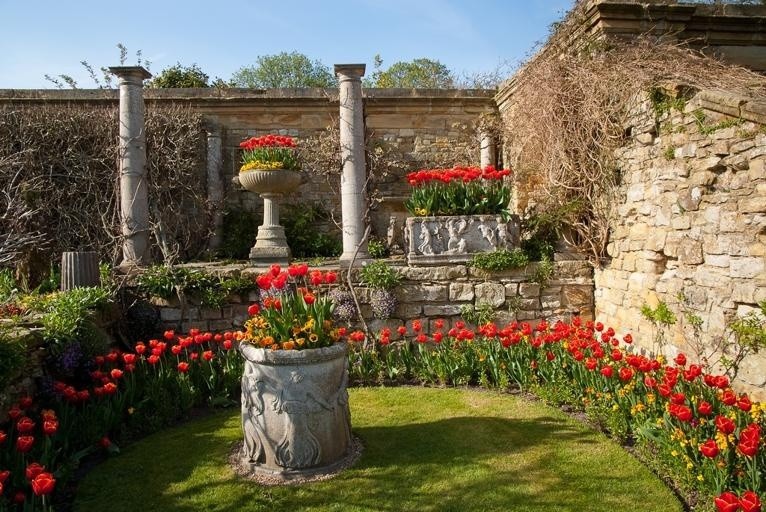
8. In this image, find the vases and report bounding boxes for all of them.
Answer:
[239,339,353,478]
[238,170,302,198]
[406,213,521,267]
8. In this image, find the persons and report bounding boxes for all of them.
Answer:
[384,216,397,250]
[418,217,514,254]
[241,362,263,463]
[275,370,334,467]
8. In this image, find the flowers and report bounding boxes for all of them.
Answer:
[239,133,304,172]
[402,162,514,221]
[235,262,347,348]
